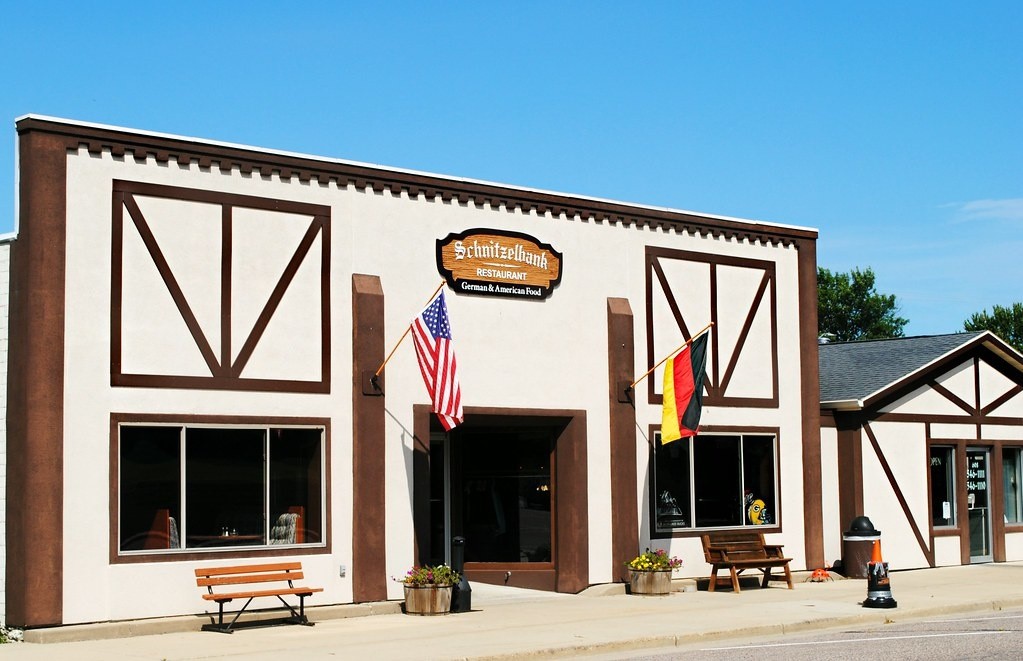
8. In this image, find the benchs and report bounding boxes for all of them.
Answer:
[194,563,324,631]
[702,529,792,593]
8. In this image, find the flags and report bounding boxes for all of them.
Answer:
[661,330,709,445]
[411,288,464,432]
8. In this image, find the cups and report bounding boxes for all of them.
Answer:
[230,528,238,535]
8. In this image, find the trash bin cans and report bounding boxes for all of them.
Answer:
[843,517,882,578]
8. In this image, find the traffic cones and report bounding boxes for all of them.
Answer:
[870,542,882,564]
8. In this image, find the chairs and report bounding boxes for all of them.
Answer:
[146,508,179,550]
[268,506,307,546]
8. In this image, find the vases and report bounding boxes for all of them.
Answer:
[628,567,672,594]
[405,582,451,614]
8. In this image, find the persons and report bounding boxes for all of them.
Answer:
[745,490,769,525]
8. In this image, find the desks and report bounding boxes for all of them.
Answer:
[188,535,266,547]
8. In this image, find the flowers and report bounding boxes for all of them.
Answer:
[625,549,683,575]
[402,563,460,586]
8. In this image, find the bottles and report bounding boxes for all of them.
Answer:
[221,527,230,537]
